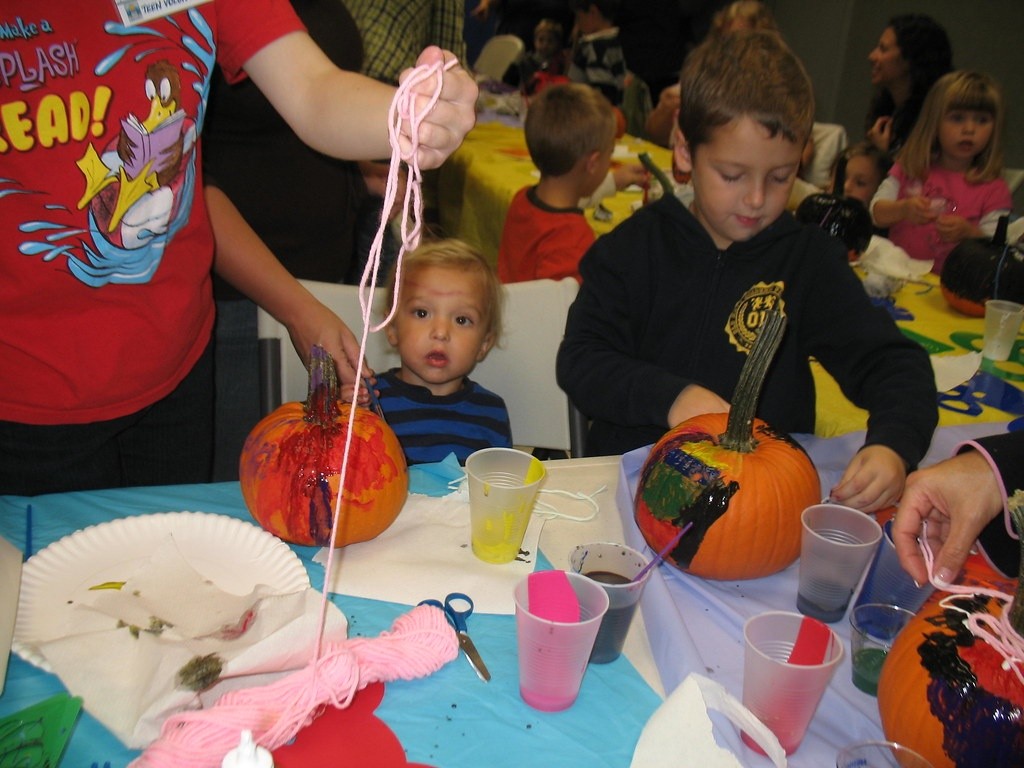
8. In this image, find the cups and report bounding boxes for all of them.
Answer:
[835,742,934,768]
[796,504,883,625]
[853,517,936,641]
[569,544,650,664]
[981,300,1024,362]
[512,571,610,712]
[740,610,844,759]
[466,448,545,564]
[848,603,916,697]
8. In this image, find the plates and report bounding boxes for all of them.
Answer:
[9,510,312,673]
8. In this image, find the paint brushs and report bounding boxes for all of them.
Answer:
[631,520,695,582]
[364,378,385,421]
[26,503,33,561]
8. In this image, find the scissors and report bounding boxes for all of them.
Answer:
[415,592,491,683]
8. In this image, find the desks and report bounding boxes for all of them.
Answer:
[432,113,1024,426]
[0,424,1024,768]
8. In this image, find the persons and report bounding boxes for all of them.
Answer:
[831,0,956,205]
[891,429,1024,589]
[342,0,473,239]
[0,0,478,496]
[868,70,1014,278]
[201,0,421,484]
[645,1,816,178]
[556,24,940,513]
[364,241,513,466]
[496,82,617,288]
[463,0,625,106]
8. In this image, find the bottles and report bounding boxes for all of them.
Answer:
[221,729,274,768]
